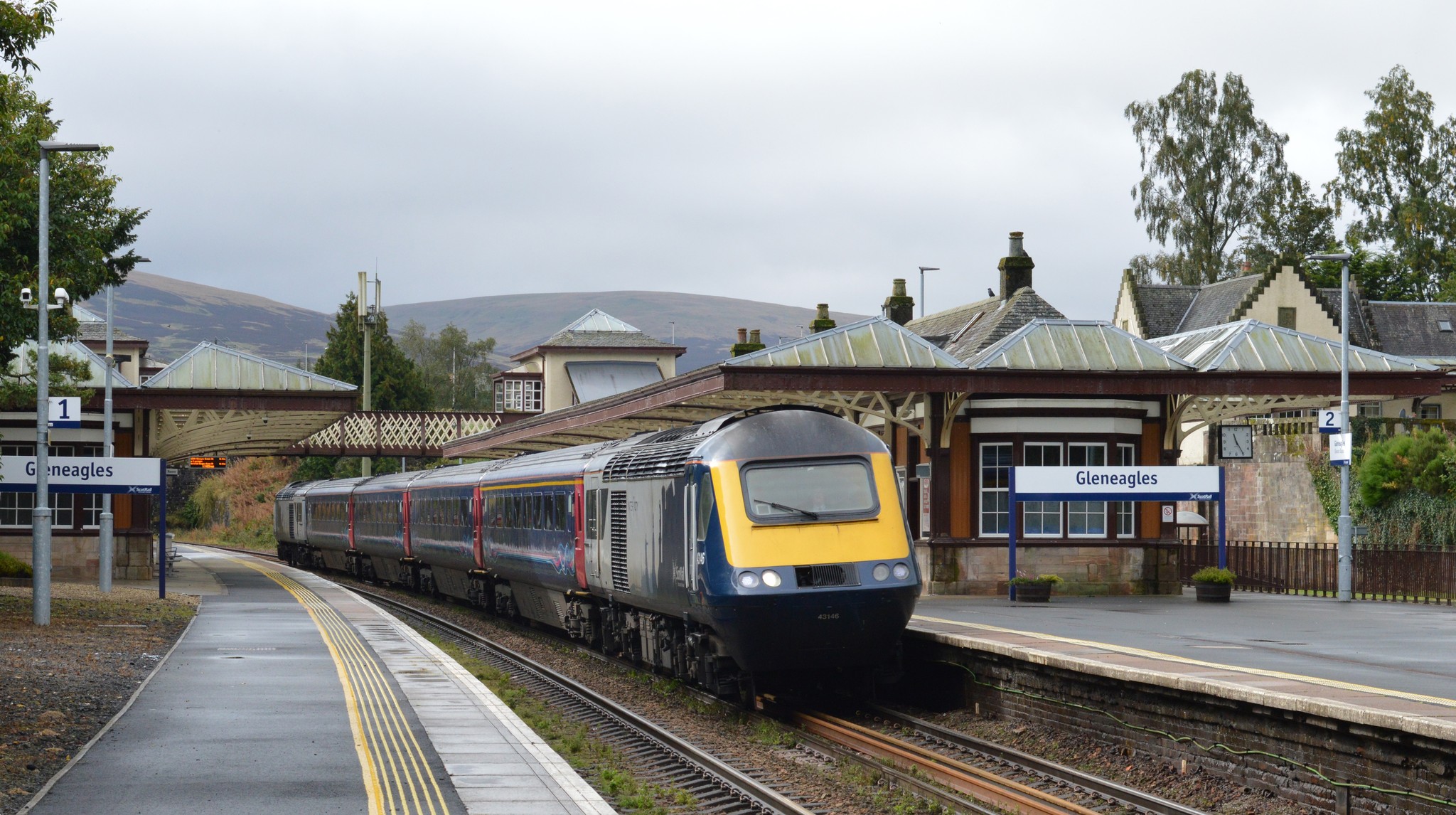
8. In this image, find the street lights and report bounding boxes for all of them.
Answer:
[916,266,941,319]
[95,254,156,590]
[1301,250,1367,606]
[18,140,104,626]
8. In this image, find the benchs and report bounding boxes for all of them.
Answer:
[155,533,183,578]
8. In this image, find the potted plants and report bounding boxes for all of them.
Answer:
[1002,569,1065,603]
[1190,566,1238,603]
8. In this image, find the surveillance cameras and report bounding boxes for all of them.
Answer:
[19,288,39,311]
[46,287,69,312]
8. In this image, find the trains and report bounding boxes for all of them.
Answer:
[273,400,950,703]
